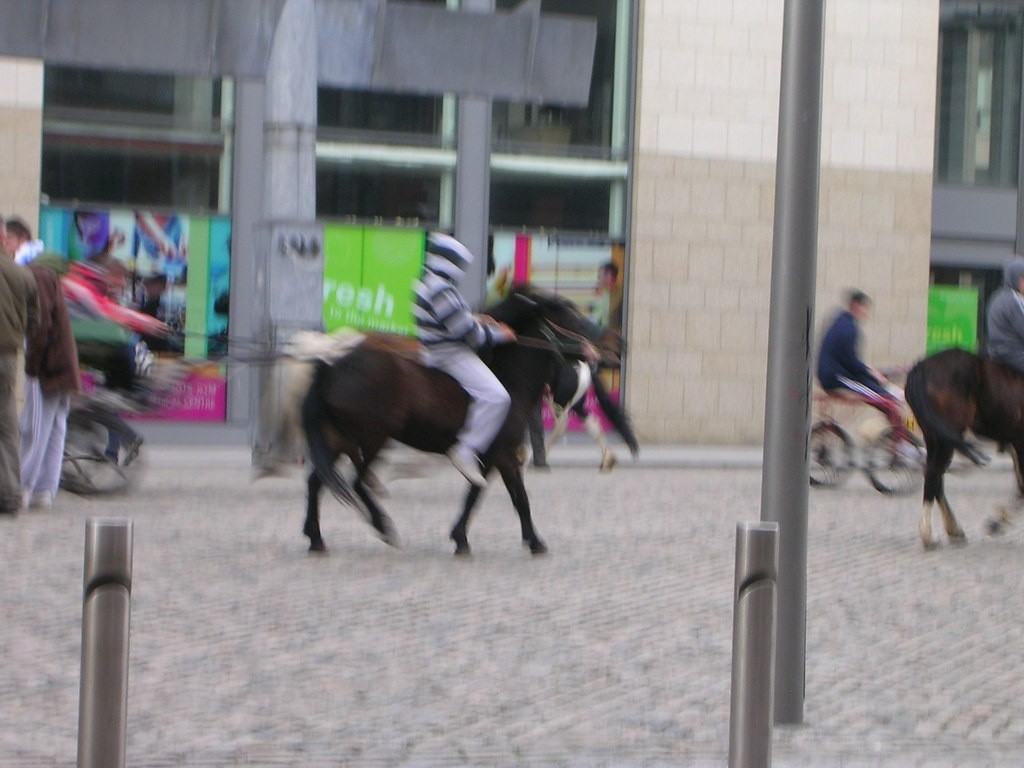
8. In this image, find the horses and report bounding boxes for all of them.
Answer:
[291,292,640,561]
[902,345,1024,548]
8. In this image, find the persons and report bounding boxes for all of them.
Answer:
[986,258,1024,370]
[814,291,910,450]
[411,231,515,488]
[592,261,623,332]
[0,219,171,513]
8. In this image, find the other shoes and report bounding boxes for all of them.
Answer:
[447,445,487,489]
[125,436,142,465]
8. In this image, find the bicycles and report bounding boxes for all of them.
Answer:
[805,389,929,498]
